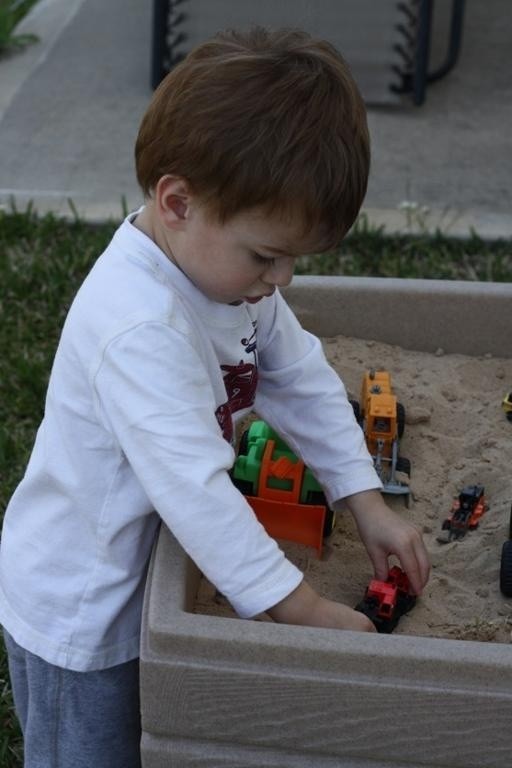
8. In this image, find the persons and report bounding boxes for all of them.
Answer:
[1,24,430,768]
